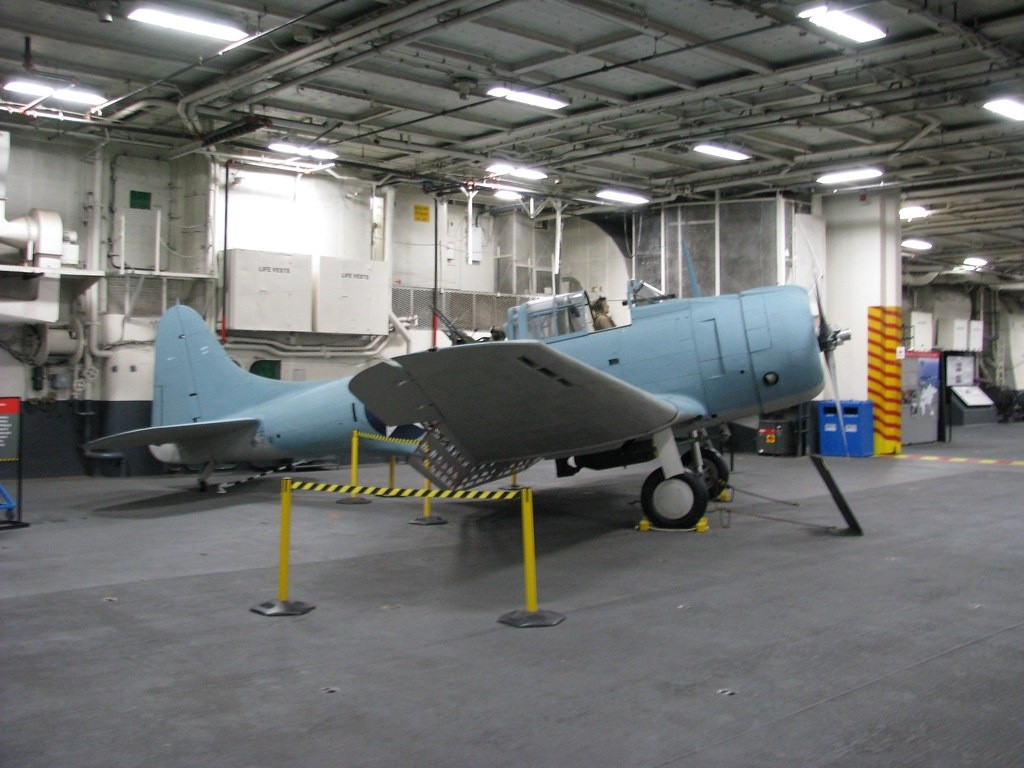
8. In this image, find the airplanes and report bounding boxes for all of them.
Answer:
[85,238,853,540]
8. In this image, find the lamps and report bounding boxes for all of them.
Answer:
[0,0,1024,275]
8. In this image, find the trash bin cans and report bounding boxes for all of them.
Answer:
[817,399,874,458]
[756,402,810,455]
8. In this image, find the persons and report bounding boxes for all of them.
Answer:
[593,296,616,331]
